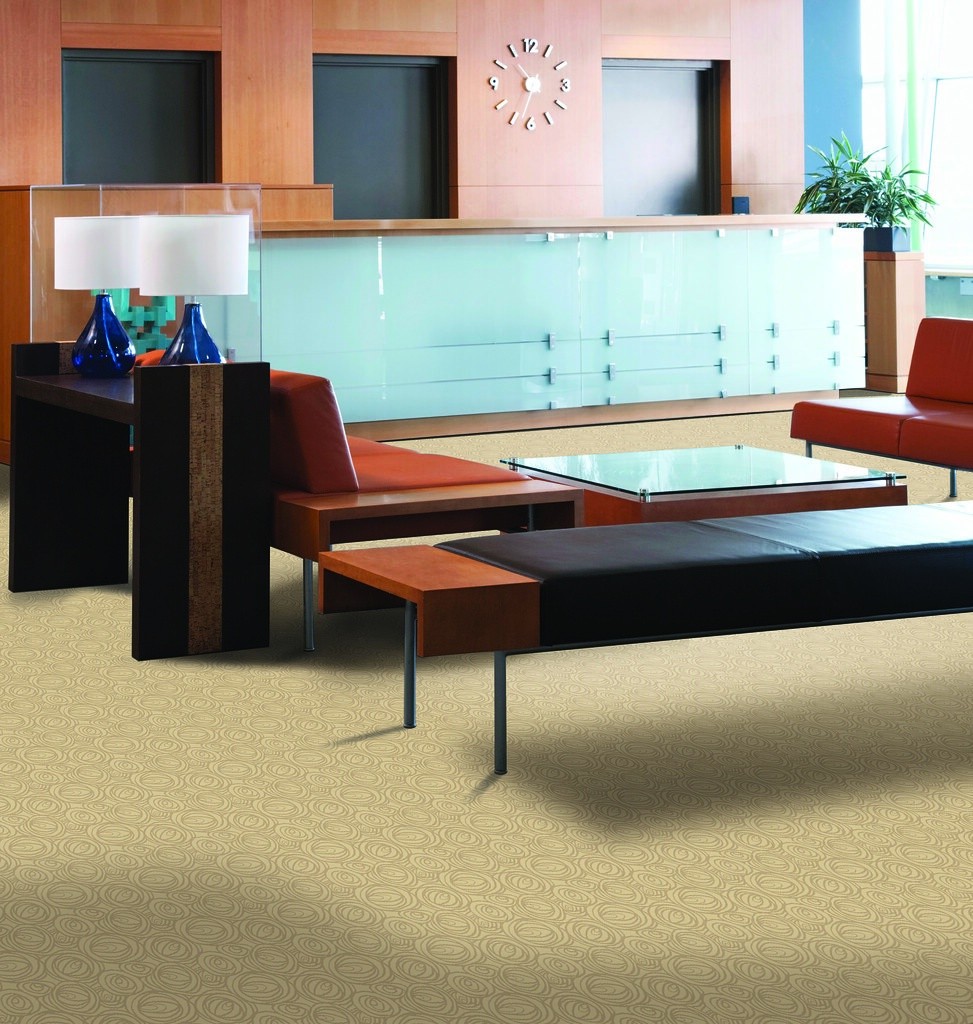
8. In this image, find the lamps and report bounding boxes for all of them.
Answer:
[54,215,137,378]
[136,216,250,364]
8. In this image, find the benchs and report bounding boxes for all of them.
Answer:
[791,318,973,495]
[316,501,973,776]
[272,370,577,652]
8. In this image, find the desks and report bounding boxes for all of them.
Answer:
[9,342,270,660]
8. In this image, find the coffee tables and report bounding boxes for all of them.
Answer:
[498,446,910,540]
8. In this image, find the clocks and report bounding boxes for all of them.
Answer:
[489,37,573,132]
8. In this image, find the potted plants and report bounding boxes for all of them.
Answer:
[798,135,937,250]
